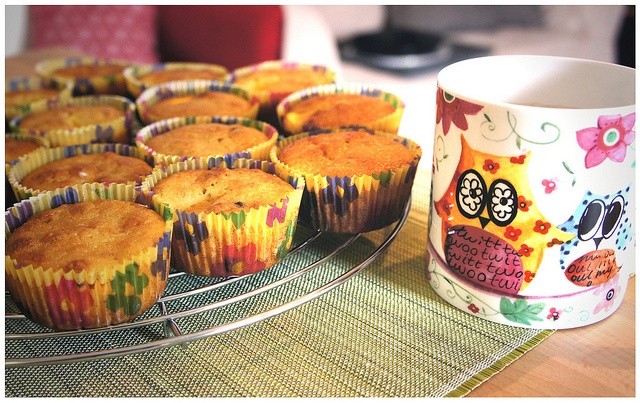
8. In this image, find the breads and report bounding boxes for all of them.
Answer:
[19,150,154,192]
[144,123,271,159]
[146,92,254,119]
[231,66,335,92]
[137,69,222,86]
[5,56,33,80]
[282,92,397,129]
[46,64,125,80]
[275,130,418,179]
[18,105,126,130]
[5,140,39,162]
[5,89,61,105]
[5,199,167,275]
[150,166,297,215]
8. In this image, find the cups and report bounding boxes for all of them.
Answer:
[423,54,635,329]
